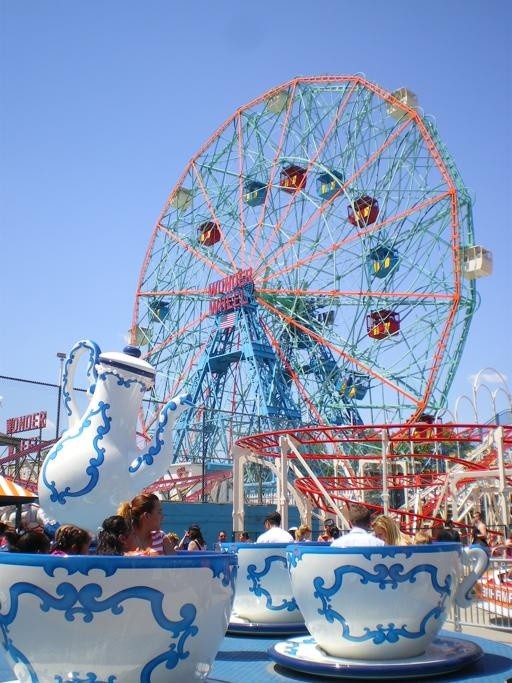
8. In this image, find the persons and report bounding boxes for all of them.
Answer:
[217,503,493,547]
[0,493,205,555]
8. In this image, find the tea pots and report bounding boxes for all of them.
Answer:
[36,337,194,534]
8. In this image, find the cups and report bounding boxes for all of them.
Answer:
[217,540,331,624]
[284,541,488,661]
[1,551,240,682]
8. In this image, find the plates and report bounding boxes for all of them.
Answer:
[226,612,310,636]
[270,639,484,683]
